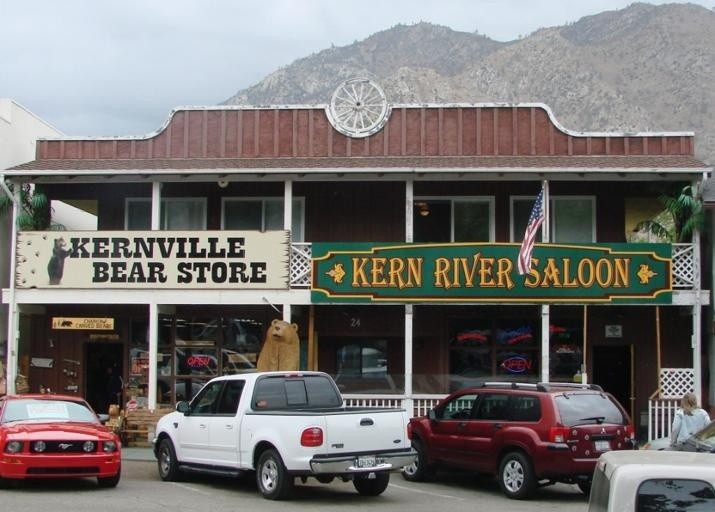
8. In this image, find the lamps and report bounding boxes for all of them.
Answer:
[414,202,430,217]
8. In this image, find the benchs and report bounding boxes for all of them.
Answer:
[120,408,174,447]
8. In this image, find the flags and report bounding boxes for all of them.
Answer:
[516,182,546,275]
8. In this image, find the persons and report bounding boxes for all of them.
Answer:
[670,392,715,451]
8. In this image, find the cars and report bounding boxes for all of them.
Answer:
[587,419,715,512]
[1,394,122,486]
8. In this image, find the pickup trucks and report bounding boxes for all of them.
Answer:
[151,370,419,501]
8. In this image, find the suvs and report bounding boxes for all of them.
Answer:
[401,381,635,499]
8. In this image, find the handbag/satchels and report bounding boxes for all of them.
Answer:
[671,421,688,447]
